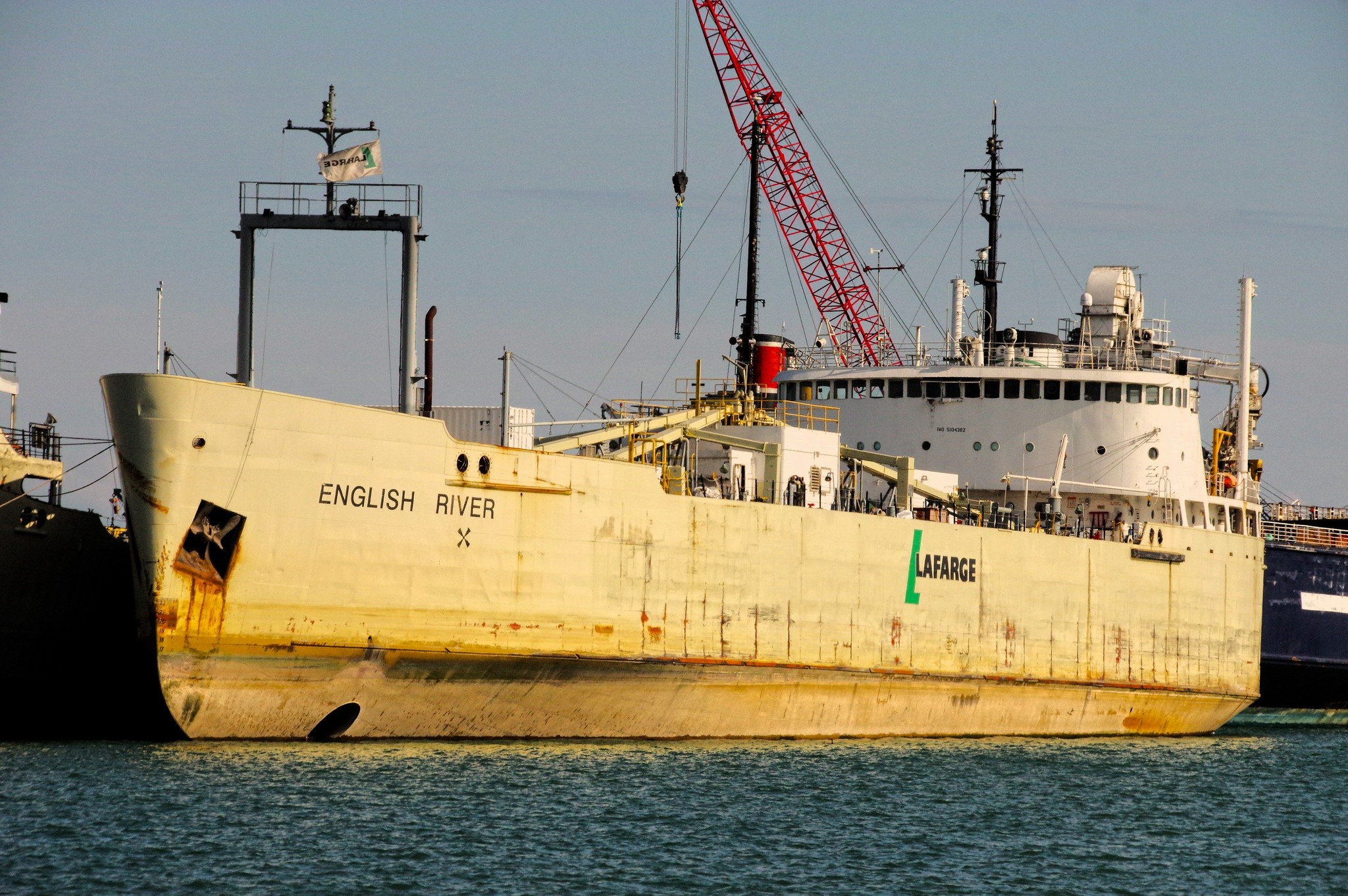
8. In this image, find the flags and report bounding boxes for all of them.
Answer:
[317,138,382,182]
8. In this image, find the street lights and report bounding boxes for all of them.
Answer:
[869,248,884,367]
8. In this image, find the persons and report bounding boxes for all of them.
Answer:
[1127,526,1133,544]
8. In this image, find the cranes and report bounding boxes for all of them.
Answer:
[696,0,905,366]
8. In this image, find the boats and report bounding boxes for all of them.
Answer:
[95,84,1266,742]
[0,288,188,742]
[1153,340,1348,709]
